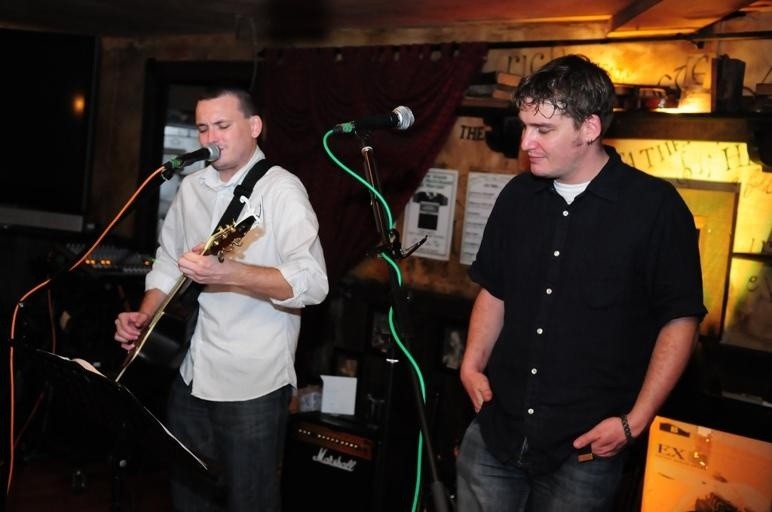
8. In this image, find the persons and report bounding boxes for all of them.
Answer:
[110,79,332,510]
[452,52,710,511]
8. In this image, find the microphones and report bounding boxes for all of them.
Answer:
[163,142,221,177]
[333,105,416,136]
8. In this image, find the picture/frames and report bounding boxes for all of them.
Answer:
[399,166,460,262]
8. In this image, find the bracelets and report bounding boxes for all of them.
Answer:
[620,414,636,444]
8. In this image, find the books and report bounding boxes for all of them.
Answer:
[614,80,681,112]
[462,67,523,101]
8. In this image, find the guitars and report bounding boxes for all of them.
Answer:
[115,216,256,382]
